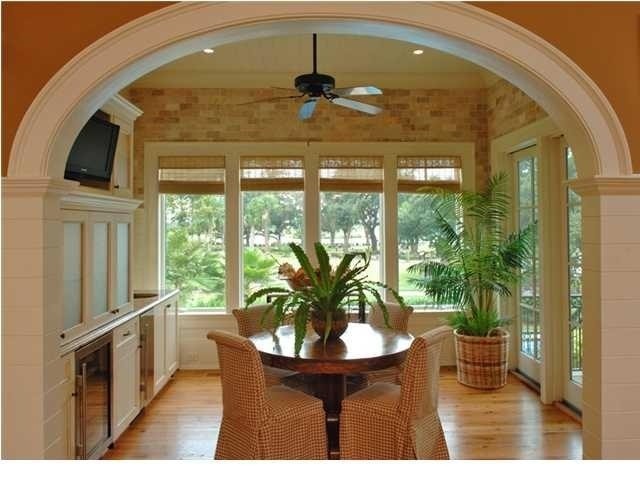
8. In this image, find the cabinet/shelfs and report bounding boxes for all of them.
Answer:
[114,318,142,437]
[155,295,180,388]
[90,209,134,332]
[61,209,89,346]
[60,353,78,460]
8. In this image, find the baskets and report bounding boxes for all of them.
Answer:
[453,326,509,389]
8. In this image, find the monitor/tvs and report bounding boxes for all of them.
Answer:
[65,116,121,183]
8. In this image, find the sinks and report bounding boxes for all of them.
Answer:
[133,292,159,298]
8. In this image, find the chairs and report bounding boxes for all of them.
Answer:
[206,301,455,459]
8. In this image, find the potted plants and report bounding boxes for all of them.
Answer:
[404,170,540,390]
[244,241,405,356]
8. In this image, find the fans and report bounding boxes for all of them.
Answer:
[235,33,385,121]
